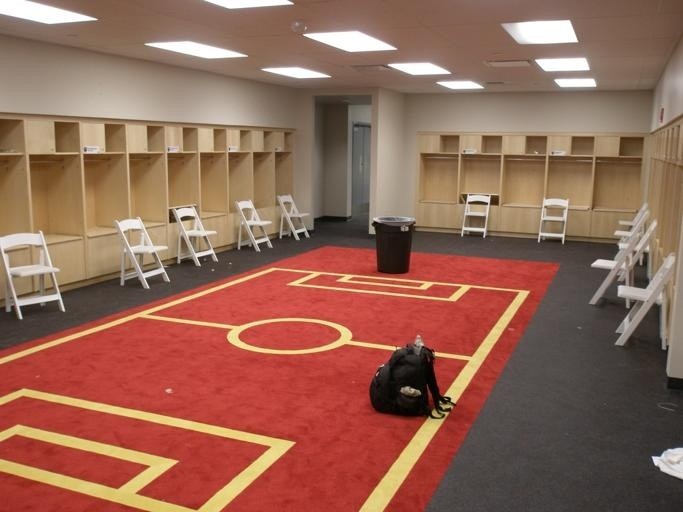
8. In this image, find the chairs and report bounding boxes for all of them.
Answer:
[615,203,658,275]
[276,194,310,241]
[615,252,677,351]
[591,221,658,308]
[0,230,66,320]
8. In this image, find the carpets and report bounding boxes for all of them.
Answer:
[0,246,560,512]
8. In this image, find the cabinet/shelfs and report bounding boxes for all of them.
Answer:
[0,112,295,307]
[648,115,683,377]
[414,131,651,246]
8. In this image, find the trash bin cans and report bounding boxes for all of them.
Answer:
[372,216,415,273]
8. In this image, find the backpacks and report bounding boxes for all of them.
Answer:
[369,345,457,419]
